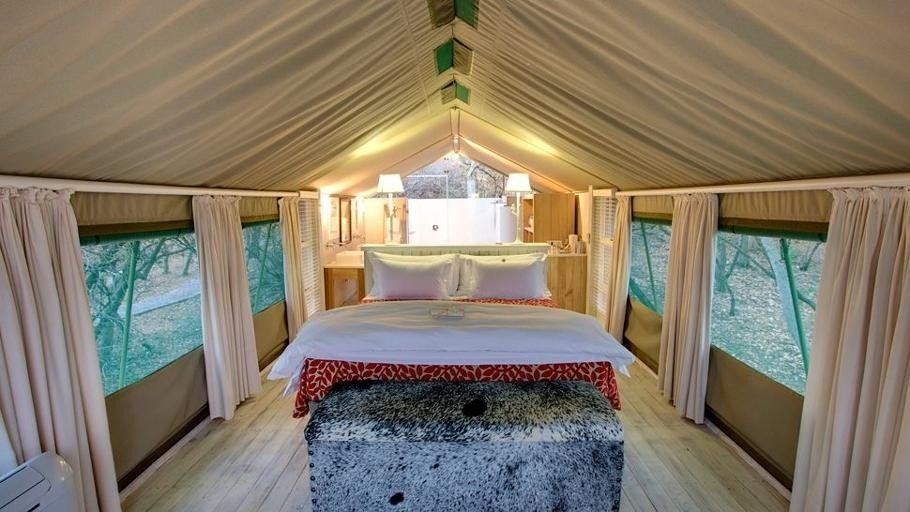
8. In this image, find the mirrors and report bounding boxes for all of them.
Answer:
[339,197,352,245]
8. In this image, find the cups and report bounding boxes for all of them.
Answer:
[568,234,585,254]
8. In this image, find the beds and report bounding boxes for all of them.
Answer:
[267,245,637,419]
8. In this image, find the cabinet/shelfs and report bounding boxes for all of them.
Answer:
[521,195,576,250]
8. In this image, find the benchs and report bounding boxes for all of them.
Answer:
[303,380,625,512]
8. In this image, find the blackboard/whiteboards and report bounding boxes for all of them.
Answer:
[406,198,498,245]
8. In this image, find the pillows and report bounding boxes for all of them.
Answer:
[360,251,553,301]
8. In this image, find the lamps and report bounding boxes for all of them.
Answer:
[375,174,405,246]
[504,172,533,246]
